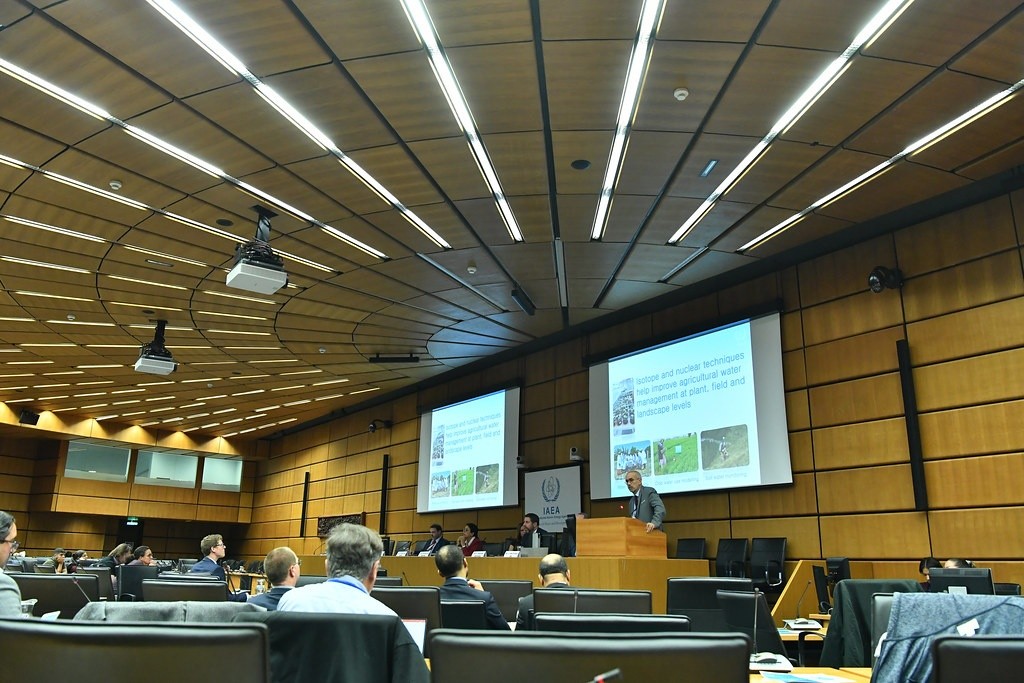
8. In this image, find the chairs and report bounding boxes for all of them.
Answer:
[388,538,562,556]
[0,555,1024,683]
[676,537,786,595]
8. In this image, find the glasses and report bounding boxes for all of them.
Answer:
[3,540,21,549]
[148,554,153,557]
[293,559,301,565]
[215,544,224,546]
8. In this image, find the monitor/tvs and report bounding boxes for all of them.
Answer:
[824,558,851,598]
[929,567,995,595]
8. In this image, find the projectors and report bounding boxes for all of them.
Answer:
[135,358,177,375]
[225,263,288,295]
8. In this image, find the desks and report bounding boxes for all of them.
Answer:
[809,613,831,628]
[749,667,870,683]
[296,554,709,615]
[225,572,249,592]
[777,627,828,641]
[248,573,271,595]
[839,667,873,679]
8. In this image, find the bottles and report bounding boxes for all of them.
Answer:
[256,580,265,594]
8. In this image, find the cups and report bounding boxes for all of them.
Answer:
[20,599,39,615]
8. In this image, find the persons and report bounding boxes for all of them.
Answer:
[657,438,666,469]
[626,471,666,533]
[616,449,647,475]
[719,436,728,460]
[248,547,302,611]
[509,513,551,554]
[0,511,21,616]
[515,553,570,630]
[277,522,398,615]
[190,534,249,602]
[43,543,153,594]
[919,557,976,592]
[435,545,511,630]
[568,513,588,556]
[423,524,450,556]
[457,523,482,556]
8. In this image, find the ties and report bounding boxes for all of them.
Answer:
[429,538,438,550]
[631,495,638,518]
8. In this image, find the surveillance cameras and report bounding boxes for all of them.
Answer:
[517,456,525,462]
[747,587,777,663]
[570,447,577,454]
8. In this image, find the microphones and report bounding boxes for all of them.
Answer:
[402,571,411,587]
[78,564,88,574]
[157,561,162,567]
[182,560,188,572]
[393,540,411,556]
[223,560,238,598]
[574,591,579,613]
[72,577,92,602]
[15,556,24,572]
[314,543,325,555]
[33,563,44,573]
[258,570,273,589]
[589,669,624,683]
[794,580,812,624]
[477,536,487,551]
